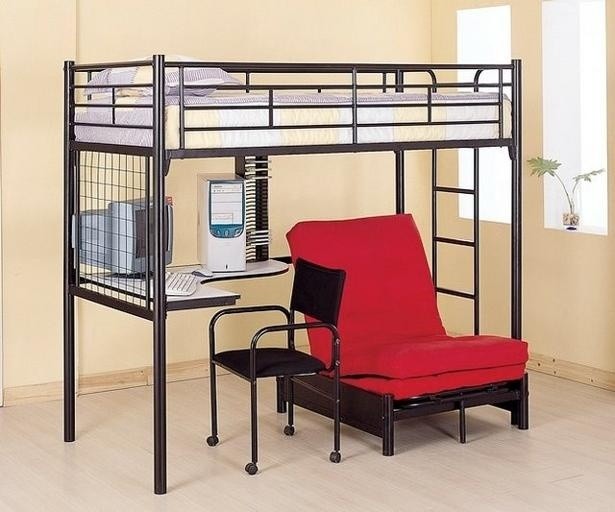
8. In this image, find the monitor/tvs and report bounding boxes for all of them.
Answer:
[78,196,174,282]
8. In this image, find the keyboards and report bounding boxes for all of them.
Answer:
[163,270,199,295]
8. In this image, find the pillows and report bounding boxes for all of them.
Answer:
[87,54,240,97]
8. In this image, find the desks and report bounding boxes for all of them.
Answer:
[63,259,291,492]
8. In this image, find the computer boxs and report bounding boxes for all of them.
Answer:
[196,172,247,272]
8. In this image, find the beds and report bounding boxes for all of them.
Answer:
[63,52,524,161]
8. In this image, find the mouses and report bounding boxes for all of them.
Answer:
[193,266,213,278]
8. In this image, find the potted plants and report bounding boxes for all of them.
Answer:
[527,154,606,229]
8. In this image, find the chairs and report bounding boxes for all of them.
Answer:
[207,213,528,475]
